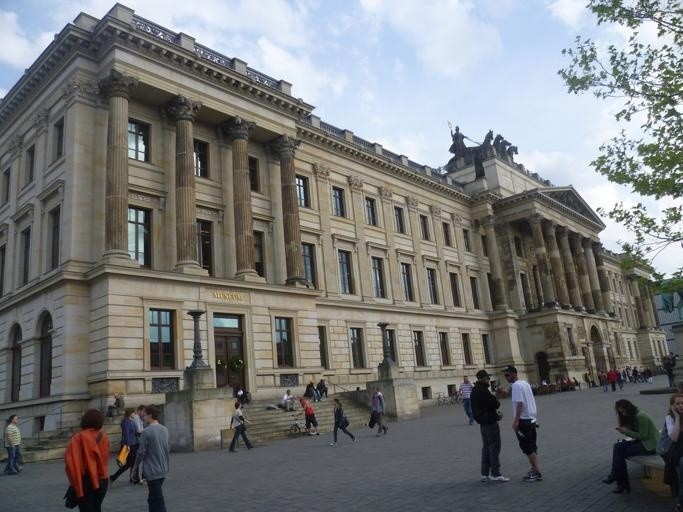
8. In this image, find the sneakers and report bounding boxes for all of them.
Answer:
[383,427,387,434]
[490,475,509,481]
[479,475,491,482]
[329,442,336,446]
[523,473,542,482]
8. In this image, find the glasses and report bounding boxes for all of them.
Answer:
[504,374,511,377]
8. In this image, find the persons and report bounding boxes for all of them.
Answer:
[3,414,23,474]
[371,385,389,437]
[657,394,683,497]
[470,370,511,482]
[448,126,468,156]
[459,375,475,425]
[228,401,255,453]
[493,350,678,398]
[281,379,328,436]
[500,365,544,482]
[328,399,357,446]
[602,400,659,495]
[64,391,171,512]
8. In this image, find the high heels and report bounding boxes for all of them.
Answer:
[612,485,630,495]
[602,474,617,485]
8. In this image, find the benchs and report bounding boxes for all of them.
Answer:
[628,454,672,497]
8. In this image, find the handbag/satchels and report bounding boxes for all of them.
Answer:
[340,416,349,429]
[116,443,131,468]
[63,485,78,509]
[655,421,673,456]
[368,415,376,429]
[241,424,246,431]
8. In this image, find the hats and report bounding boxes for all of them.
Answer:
[476,370,492,379]
[500,365,516,373]
